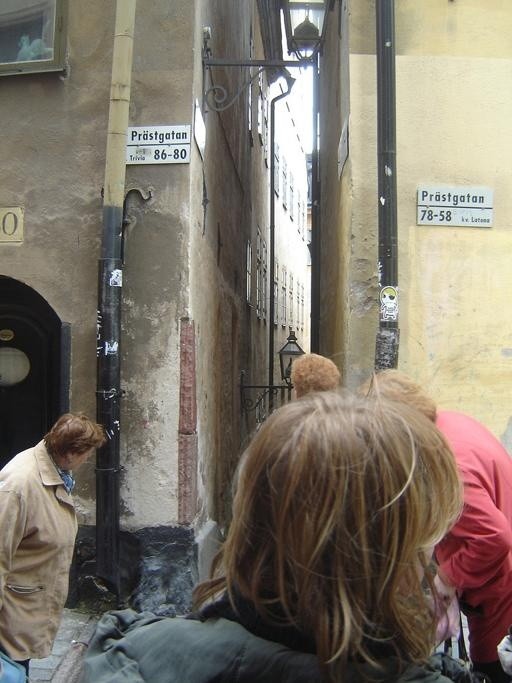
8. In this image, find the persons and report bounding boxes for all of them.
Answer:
[289,353,341,399]
[0,413,107,681]
[77,383,478,682]
[362,365,512,683]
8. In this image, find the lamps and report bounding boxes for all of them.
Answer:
[238,330,307,415]
[201,0,334,115]
